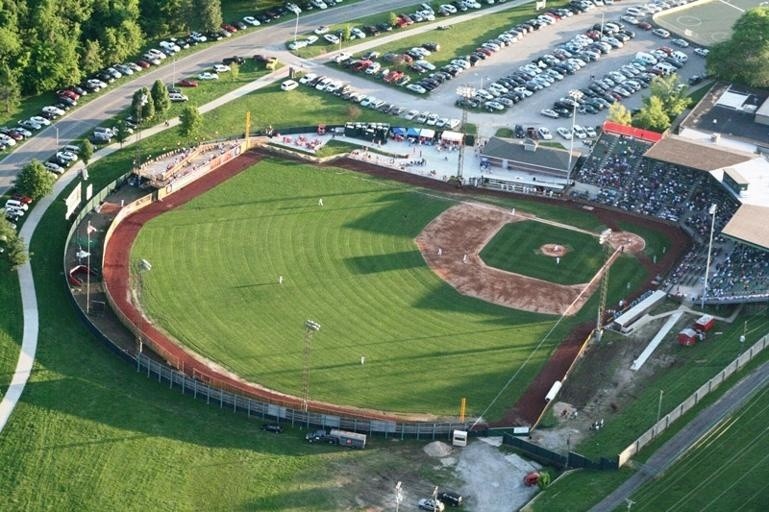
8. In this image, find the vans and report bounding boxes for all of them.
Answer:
[438,491,463,506]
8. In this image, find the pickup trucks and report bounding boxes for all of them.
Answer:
[305,428,338,445]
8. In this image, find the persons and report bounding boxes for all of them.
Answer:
[579,132,768,298]
[619,298,626,310]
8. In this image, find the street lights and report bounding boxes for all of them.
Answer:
[302,317,320,420]
[136,257,151,372]
[699,201,716,307]
[597,226,612,327]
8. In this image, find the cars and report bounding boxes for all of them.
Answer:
[260,422,284,434]
[419,497,445,512]
[0,0,712,243]
